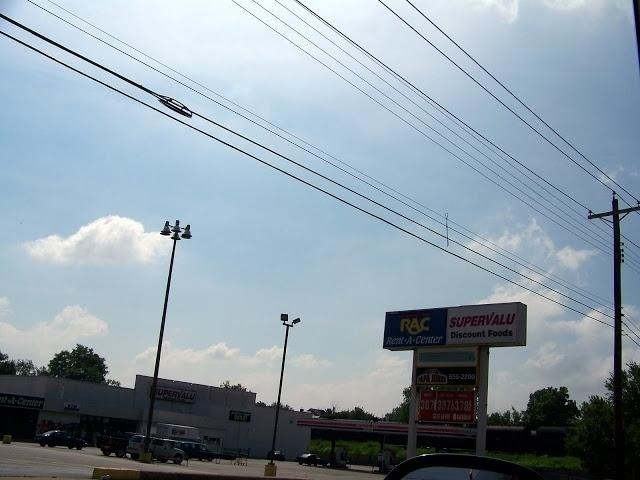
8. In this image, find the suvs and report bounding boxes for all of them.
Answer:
[99,421,221,467]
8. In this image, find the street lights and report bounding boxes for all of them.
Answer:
[263,306,303,478]
[136,218,200,458]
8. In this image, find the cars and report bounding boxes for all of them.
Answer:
[267,447,286,462]
[34,429,87,452]
[295,453,329,468]
